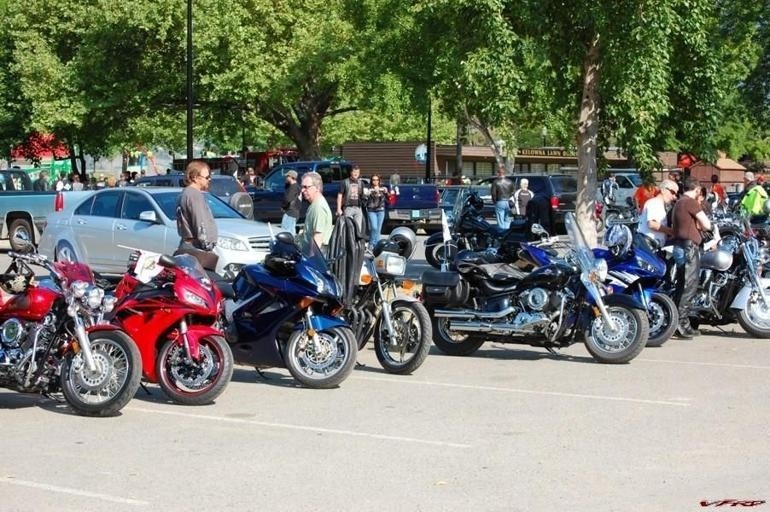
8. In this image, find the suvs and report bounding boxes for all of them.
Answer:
[477,174,579,234]
[129,175,254,220]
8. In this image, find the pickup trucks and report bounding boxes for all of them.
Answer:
[246,161,439,233]
[0,170,94,254]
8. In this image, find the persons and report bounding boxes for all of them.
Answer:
[491,169,515,229]
[34,170,148,191]
[698,188,712,220]
[281,170,302,237]
[634,181,657,214]
[390,168,400,185]
[668,177,710,340]
[300,173,332,258]
[336,166,363,234]
[710,174,728,206]
[601,173,618,206]
[514,179,535,215]
[176,162,218,253]
[637,179,679,249]
[365,175,386,249]
[741,172,767,214]
[239,168,257,188]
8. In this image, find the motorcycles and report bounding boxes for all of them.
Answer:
[204,233,357,387]
[95,245,233,405]
[423,184,542,268]
[337,214,433,374]
[651,197,770,336]
[0,233,144,416]
[515,197,679,347]
[423,211,649,364]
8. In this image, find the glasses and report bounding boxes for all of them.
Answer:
[199,175,210,180]
[300,185,312,189]
[665,187,677,196]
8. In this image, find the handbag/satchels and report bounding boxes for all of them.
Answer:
[509,195,516,208]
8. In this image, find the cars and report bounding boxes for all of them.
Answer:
[597,172,642,208]
[37,185,288,283]
[430,183,526,236]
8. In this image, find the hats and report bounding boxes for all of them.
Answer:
[743,172,754,181]
[284,171,298,179]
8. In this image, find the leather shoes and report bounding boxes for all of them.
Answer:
[662,319,693,340]
[679,319,701,336]
